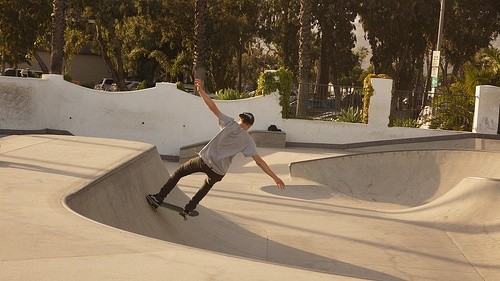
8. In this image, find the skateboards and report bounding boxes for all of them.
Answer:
[147,194,199,221]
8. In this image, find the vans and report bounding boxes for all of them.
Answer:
[4,67,45,77]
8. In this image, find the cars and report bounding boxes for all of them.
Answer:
[93,78,141,91]
[247,88,297,103]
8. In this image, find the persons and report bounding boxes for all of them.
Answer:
[149,77,286,217]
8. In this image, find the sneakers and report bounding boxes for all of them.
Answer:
[182,205,192,214]
[147,194,161,209]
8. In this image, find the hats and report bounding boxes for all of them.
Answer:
[239,112,256,125]
